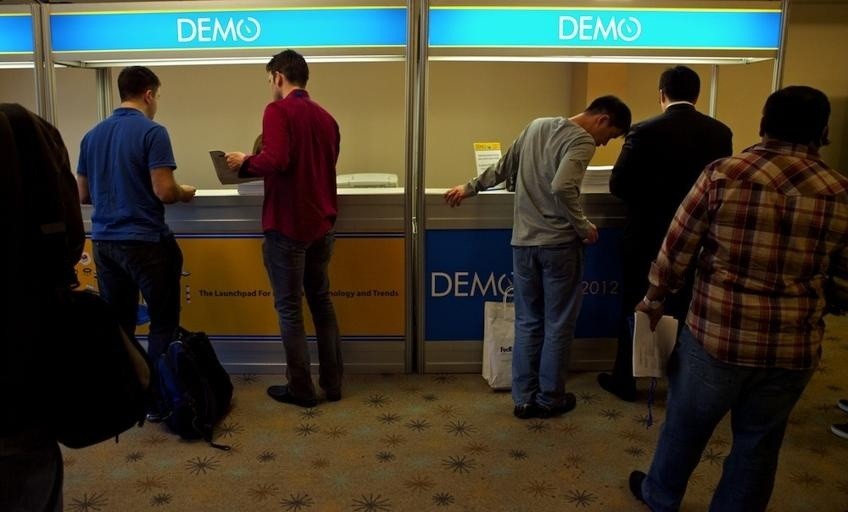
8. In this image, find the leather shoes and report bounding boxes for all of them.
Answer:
[268,386,317,408]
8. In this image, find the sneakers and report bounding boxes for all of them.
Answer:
[513,393,576,420]
[597,372,636,402]
[830,424,848,439]
[837,399,848,412]
[630,471,655,512]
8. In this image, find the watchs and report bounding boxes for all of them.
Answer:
[643,296,663,310]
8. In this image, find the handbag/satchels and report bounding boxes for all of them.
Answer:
[156,326,234,439]
[40,290,153,449]
[482,285,514,390]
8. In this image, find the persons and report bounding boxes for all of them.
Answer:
[828,398,848,438]
[77,63,197,424]
[627,84,848,511]
[595,65,734,401]
[1,100,86,512]
[443,94,632,420]
[225,48,344,408]
